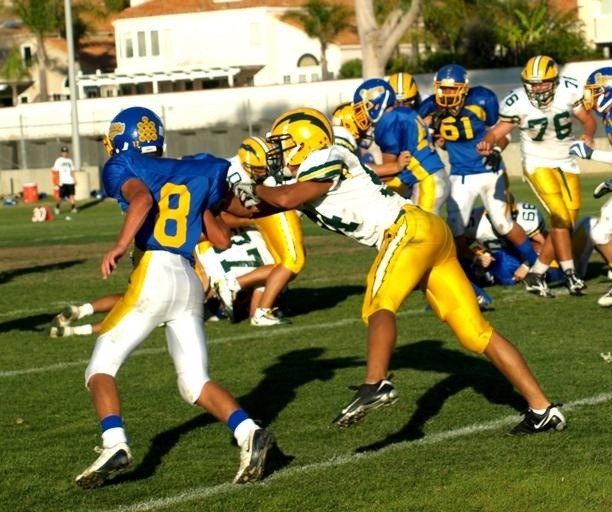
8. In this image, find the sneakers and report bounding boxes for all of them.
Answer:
[250,306,291,327]
[49,326,64,339]
[53,204,60,215]
[214,275,237,320]
[562,268,587,295]
[69,207,78,214]
[51,305,80,327]
[232,426,274,484]
[521,268,556,298]
[598,286,612,307]
[331,373,400,426]
[74,442,133,489]
[502,402,567,439]
[425,283,491,311]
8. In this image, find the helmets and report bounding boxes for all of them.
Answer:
[102,107,164,158]
[582,67,612,113]
[521,55,560,109]
[237,136,280,182]
[330,101,375,150]
[351,78,396,132]
[383,73,420,108]
[432,64,470,108]
[264,107,333,185]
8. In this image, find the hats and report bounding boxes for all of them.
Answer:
[61,146,69,152]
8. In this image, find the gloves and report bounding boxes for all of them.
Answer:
[568,141,594,160]
[593,177,612,199]
[233,181,262,208]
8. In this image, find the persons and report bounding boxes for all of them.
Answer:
[46,54,612,344]
[225,106,568,437]
[49,142,79,218]
[72,105,277,493]
